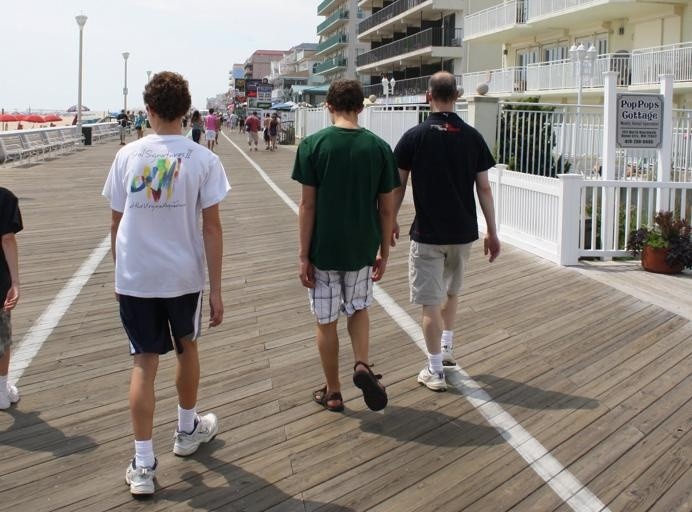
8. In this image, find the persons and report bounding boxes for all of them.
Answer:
[117,109,146,145]
[182,108,282,152]
[102,71,232,497]
[290,78,401,412]
[0,137,23,410]
[390,70,501,393]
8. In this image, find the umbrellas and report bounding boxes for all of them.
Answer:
[0,113,63,130]
[67,105,90,112]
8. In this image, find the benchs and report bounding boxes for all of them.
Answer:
[0,121,132,170]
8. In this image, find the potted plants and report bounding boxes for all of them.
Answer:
[625,207,692,275]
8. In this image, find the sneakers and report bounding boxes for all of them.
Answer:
[120,141,126,145]
[0,378,21,410]
[124,454,157,497]
[172,412,220,456]
[416,367,448,393]
[441,348,456,367]
[249,145,279,154]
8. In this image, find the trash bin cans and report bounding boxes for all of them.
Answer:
[145,119,151,128]
[82,127,91,145]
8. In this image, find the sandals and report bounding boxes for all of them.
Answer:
[312,386,344,413]
[352,361,387,412]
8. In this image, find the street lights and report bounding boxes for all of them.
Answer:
[147,70,153,83]
[299,90,303,103]
[121,51,131,110]
[74,15,88,146]
[382,77,396,110]
[570,43,596,170]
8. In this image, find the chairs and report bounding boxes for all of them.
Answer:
[610,49,633,88]
